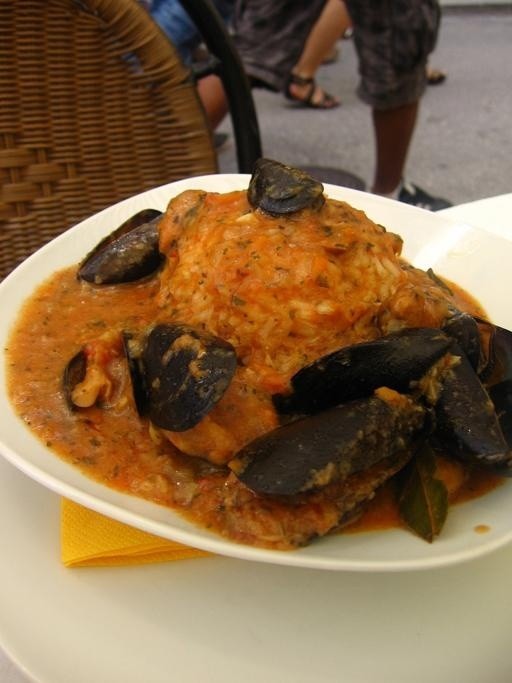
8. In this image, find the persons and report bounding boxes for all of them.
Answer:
[148,0,453,211]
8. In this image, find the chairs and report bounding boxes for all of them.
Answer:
[1,1,264,288]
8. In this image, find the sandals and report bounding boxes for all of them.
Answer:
[282,71,341,108]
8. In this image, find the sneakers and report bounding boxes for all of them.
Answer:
[377,182,453,211]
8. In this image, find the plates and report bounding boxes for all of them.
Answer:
[0,171,511,577]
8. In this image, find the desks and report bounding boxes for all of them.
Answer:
[0,188,511,683]
